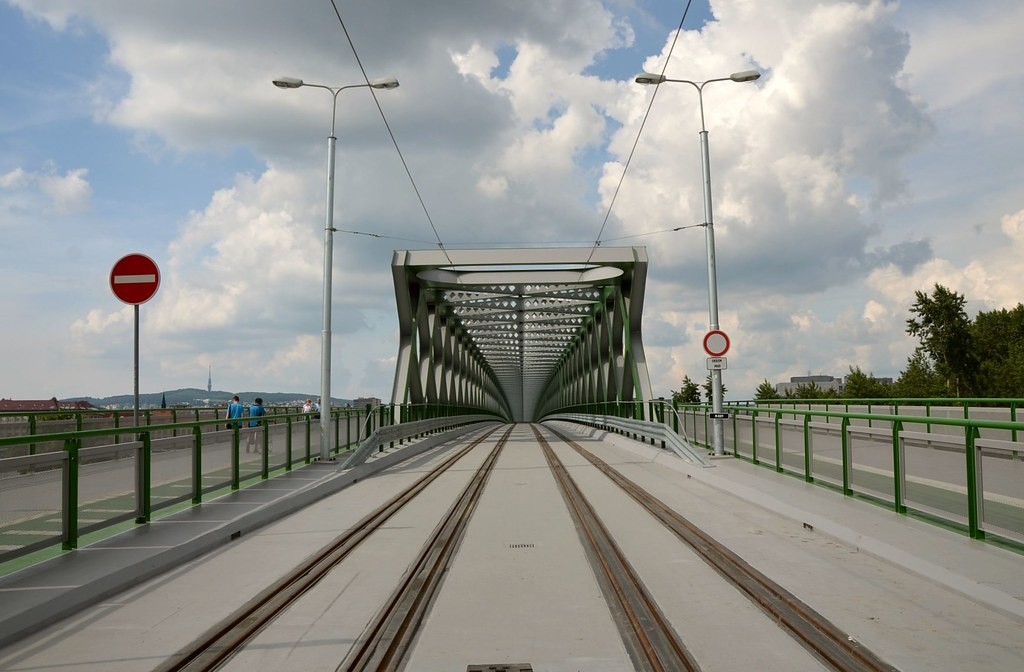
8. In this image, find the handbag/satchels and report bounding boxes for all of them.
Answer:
[226,423,232,430]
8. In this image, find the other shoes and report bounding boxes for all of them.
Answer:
[254,450,261,455]
[246,444,250,453]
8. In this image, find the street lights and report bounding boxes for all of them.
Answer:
[635,70,761,456]
[271,74,401,467]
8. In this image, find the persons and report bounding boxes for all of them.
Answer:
[245,398,266,455]
[225,396,244,444]
[302,398,321,421]
[344,401,350,410]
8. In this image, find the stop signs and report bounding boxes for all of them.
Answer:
[109,252,160,305]
